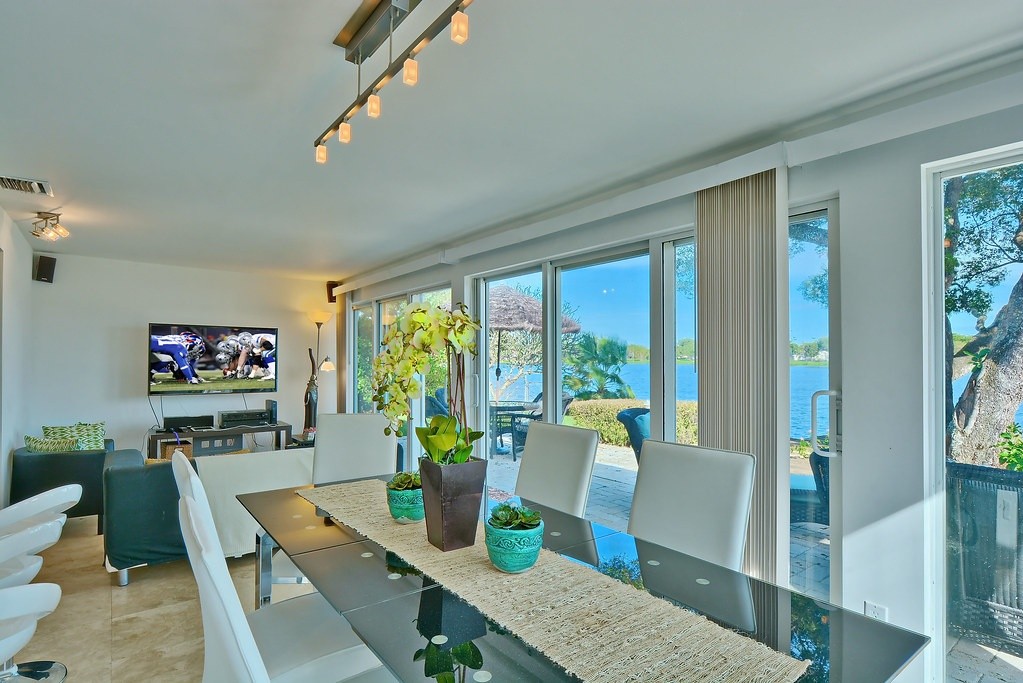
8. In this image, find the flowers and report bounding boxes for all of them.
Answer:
[372,299,481,469]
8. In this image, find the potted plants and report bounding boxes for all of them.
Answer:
[485,502,544,574]
[386,471,425,522]
[385,549,424,577]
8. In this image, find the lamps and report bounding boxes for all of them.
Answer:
[30,212,70,242]
[308,311,336,372]
[314,0,472,163]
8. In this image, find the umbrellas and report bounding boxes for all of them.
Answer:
[441,286,579,403]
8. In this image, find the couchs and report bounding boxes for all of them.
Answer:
[12,441,402,586]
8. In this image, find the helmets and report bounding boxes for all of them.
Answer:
[215,332,251,363]
[181,331,206,359]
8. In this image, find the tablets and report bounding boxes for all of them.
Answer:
[191,427,214,431]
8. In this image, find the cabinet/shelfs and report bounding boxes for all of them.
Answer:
[150,421,292,461]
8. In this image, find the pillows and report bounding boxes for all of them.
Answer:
[23,421,106,452]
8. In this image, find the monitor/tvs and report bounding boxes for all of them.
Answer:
[148,323,278,393]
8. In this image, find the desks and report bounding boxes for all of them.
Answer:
[235,472,932,683]
[490,400,539,457]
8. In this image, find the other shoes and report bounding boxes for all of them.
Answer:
[150,381,156,385]
[261,373,275,379]
[153,378,162,383]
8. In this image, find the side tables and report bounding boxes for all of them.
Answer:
[291,434,314,447]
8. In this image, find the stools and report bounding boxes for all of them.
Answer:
[0,484,82,683]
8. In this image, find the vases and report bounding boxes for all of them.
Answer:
[418,456,488,551]
[417,576,487,650]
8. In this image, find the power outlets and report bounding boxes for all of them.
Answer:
[863,601,887,621]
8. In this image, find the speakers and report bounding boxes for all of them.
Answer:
[327,283,340,303]
[36,256,56,283]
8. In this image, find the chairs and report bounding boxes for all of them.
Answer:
[521,497,600,567]
[172,387,1022,683]
[635,537,756,633]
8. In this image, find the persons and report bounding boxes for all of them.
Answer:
[151,331,276,385]
